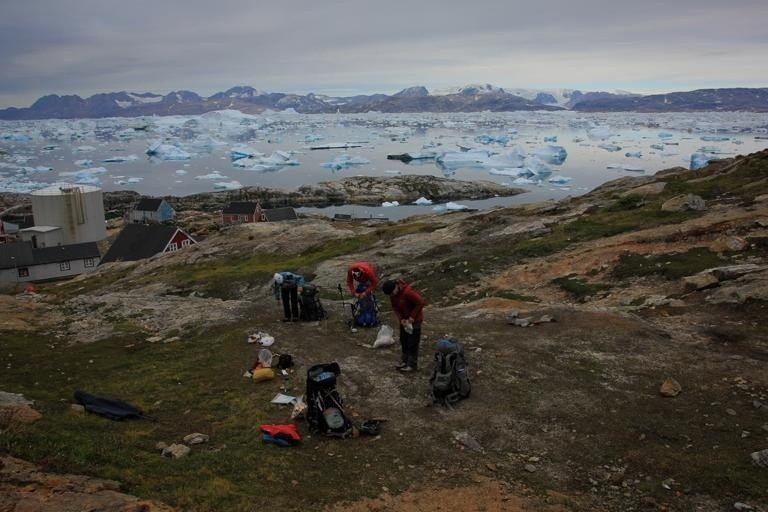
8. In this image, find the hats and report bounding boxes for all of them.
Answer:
[274,273,283,284]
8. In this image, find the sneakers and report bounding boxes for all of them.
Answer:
[395,361,415,372]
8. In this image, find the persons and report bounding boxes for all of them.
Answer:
[347,262,378,298]
[271,271,304,323]
[382,279,424,372]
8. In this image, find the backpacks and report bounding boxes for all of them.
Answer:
[299,284,328,321]
[430,340,471,404]
[353,282,375,327]
[306,362,352,438]
